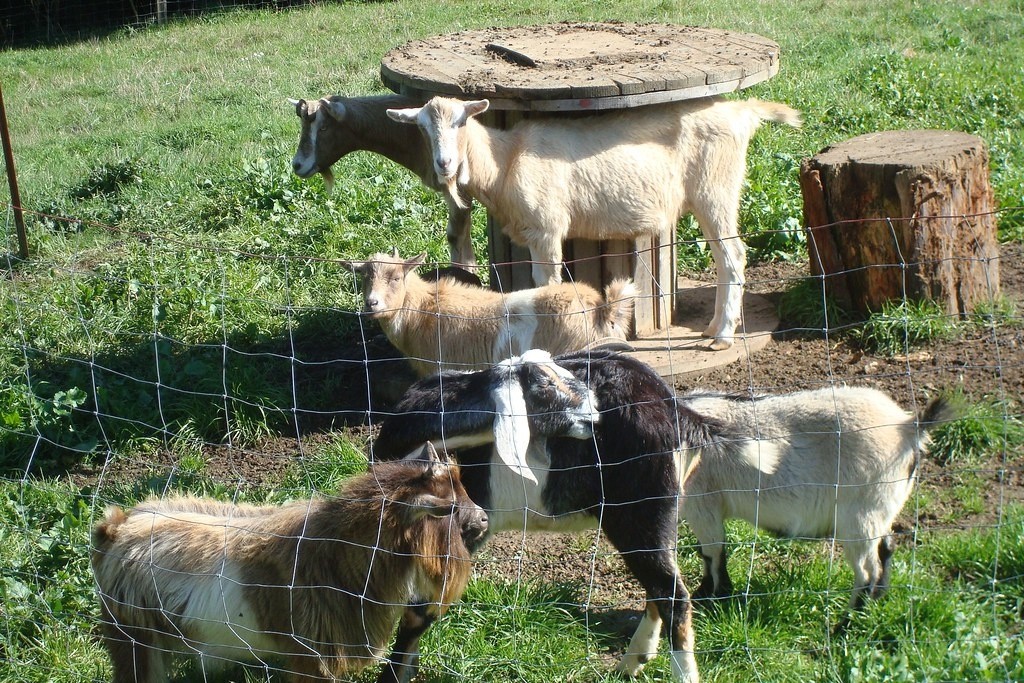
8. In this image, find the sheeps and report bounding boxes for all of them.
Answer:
[89,438,490,683]
[287,90,804,352]
[369,350,780,683]
[338,244,641,379]
[674,379,966,638]
